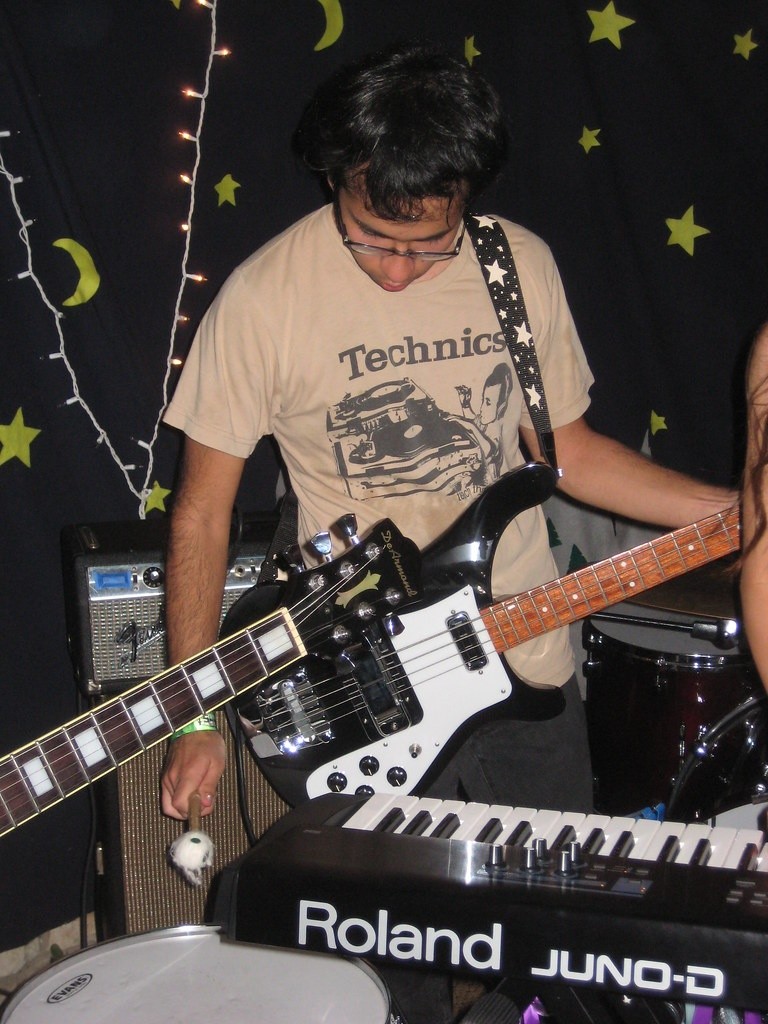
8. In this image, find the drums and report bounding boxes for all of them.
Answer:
[0,924,410,1024]
[666,686,768,830]
[582,601,768,822]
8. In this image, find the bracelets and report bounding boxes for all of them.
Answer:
[165,711,222,738]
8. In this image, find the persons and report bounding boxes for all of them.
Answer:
[150,48,743,822]
[738,314,768,721]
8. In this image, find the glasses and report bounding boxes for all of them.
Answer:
[334,186,468,261]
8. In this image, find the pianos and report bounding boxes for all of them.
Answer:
[203,792,768,1013]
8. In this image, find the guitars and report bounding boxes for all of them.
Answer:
[0,513,425,838]
[218,460,740,809]
